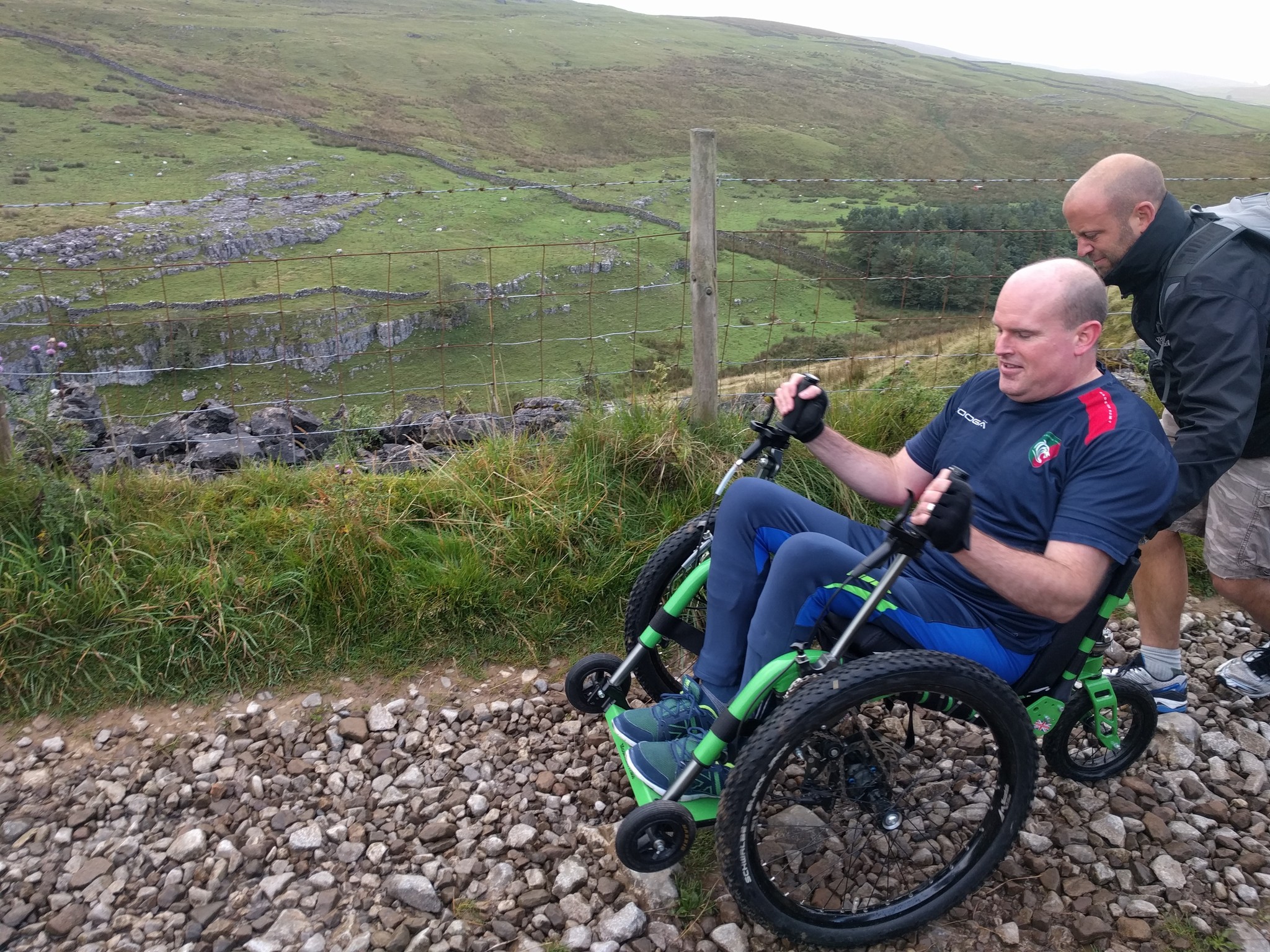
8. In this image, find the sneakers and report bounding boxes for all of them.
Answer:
[1100,653,1190,712]
[625,726,736,803]
[1213,640,1270,700]
[611,673,719,747]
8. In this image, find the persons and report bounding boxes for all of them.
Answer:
[609,256,1180,802]
[1060,153,1270,712]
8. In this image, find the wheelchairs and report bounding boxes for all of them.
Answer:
[565,373,1159,947]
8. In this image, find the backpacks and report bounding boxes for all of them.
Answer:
[1160,192,1269,321]
